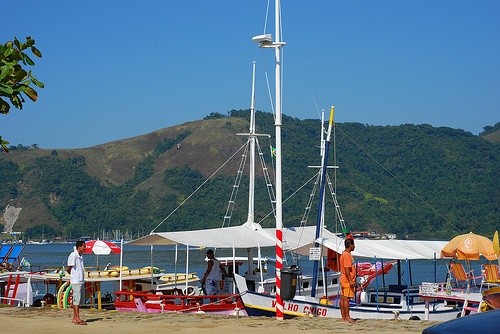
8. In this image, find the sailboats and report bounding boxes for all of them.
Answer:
[203,0,368,318]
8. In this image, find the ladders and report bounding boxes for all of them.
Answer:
[3,274,20,305]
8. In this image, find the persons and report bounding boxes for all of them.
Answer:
[67,241,88,325]
[340,239,358,323]
[200,249,229,303]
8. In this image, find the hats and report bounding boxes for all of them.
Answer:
[205,249,214,254]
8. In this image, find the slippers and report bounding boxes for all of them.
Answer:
[72,320,87,325]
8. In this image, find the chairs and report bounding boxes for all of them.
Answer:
[448,263,476,291]
[478,264,500,293]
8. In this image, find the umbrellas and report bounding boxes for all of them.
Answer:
[441,231,497,277]
[73,237,120,270]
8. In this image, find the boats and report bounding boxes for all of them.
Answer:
[231,235,498,321]
[0,263,250,317]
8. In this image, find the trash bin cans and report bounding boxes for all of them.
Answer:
[246,280,255,292]
[280,265,299,300]
